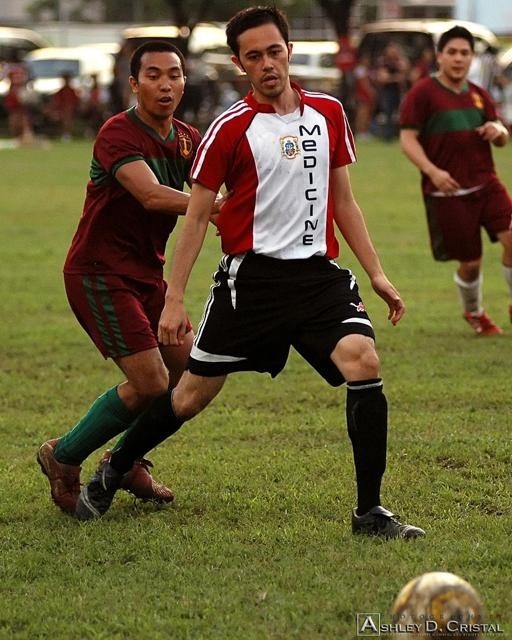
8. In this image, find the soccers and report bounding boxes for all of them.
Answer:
[390,571,485,639]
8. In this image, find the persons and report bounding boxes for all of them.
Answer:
[0,49,124,145]
[352,41,507,142]
[400,25,512,337]
[36,42,235,516]
[75,6,426,539]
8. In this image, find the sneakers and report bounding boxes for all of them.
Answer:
[351,505,426,541]
[37,437,173,520]
[463,307,502,336]
